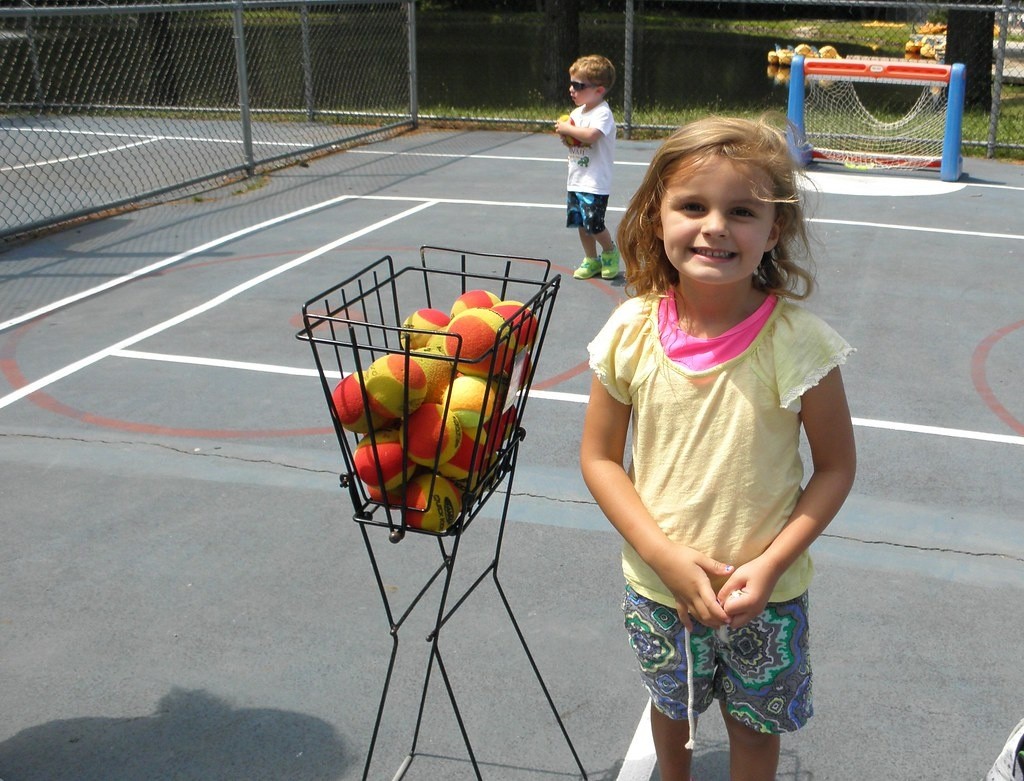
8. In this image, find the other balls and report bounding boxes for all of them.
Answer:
[557,115,582,146]
[329,287,540,533]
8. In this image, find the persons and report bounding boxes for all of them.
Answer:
[577,114,856,781]
[555,54,620,279]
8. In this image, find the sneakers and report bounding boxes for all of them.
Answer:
[601,241,619,279]
[573,255,602,280]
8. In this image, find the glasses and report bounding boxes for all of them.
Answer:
[570,81,597,91]
